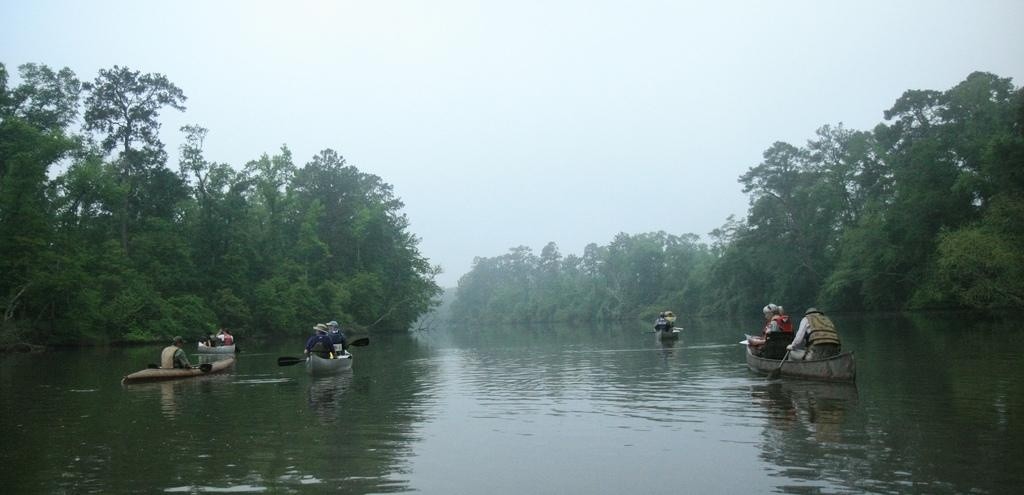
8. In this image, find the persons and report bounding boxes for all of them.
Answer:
[654,311,677,333]
[786,308,840,360]
[748,304,794,359]
[304,321,349,361]
[161,336,193,368]
[198,328,233,347]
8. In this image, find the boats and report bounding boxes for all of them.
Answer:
[197,339,237,354]
[654,326,681,342]
[301,350,354,378]
[742,333,858,385]
[122,355,236,381]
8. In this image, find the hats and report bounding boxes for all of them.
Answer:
[313,321,338,333]
[173,336,188,343]
[804,307,824,317]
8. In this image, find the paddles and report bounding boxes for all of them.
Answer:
[349,338,369,346]
[279,356,307,367]
[221,330,241,353]
[148,363,211,372]
[640,330,681,335]
[766,350,790,381]
